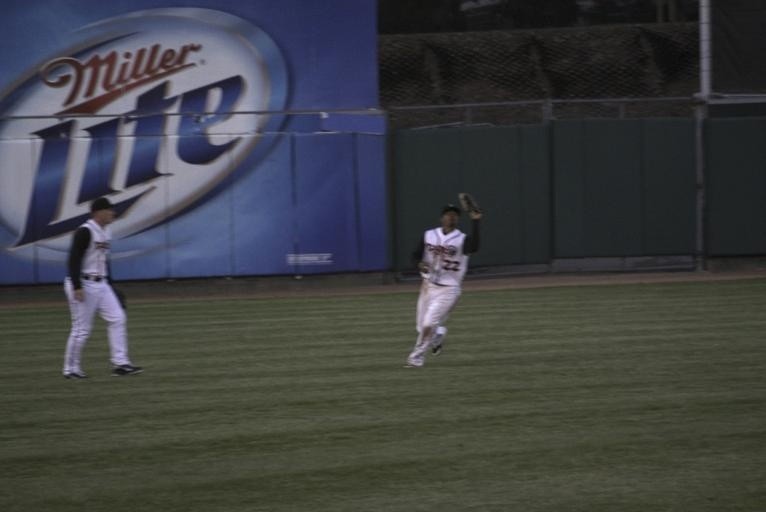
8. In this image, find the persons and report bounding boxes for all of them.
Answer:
[400,204,484,370]
[61,197,146,381]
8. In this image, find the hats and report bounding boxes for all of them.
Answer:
[440,204,461,215]
[91,198,116,212]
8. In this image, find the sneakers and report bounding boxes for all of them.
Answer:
[401,363,423,369]
[63,372,88,381]
[111,364,144,377]
[432,343,442,356]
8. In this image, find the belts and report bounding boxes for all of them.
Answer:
[81,274,102,283]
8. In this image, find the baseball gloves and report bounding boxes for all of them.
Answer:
[460,193,487,220]
